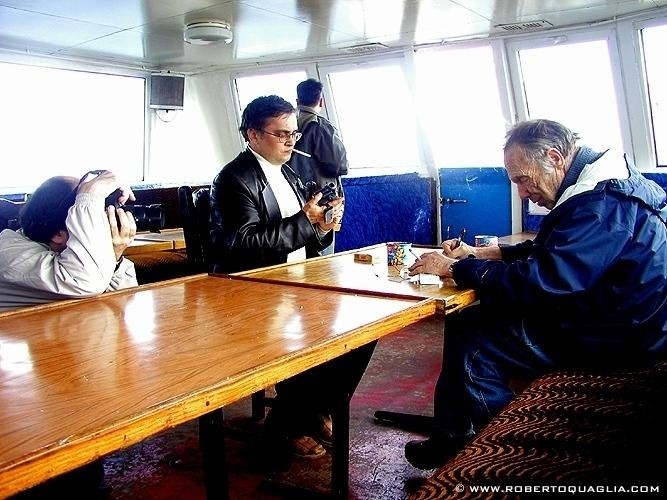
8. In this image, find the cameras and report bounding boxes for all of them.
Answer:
[311,181,338,206]
[103,190,165,234]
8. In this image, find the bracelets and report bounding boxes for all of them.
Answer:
[447,259,459,280]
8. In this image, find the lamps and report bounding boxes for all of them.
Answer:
[183,19,234,47]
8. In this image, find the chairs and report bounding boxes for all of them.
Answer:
[174,184,350,500]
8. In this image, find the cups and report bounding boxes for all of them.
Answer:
[387,241,412,266]
[475,235,499,248]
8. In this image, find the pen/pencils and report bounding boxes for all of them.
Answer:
[455,228,466,248]
[409,249,422,261]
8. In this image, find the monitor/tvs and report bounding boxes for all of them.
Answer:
[148,71,187,110]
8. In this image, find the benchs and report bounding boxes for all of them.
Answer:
[407,365,666,500]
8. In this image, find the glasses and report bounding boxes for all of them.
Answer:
[264,131,303,143]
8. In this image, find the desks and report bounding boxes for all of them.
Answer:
[229,242,476,442]
[120,239,174,269]
[135,227,187,278]
[0,272,435,500]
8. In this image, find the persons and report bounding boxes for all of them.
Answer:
[403,118,666,469]
[280,78,350,257]
[0,173,145,499]
[209,92,376,458]
[406,248,421,261]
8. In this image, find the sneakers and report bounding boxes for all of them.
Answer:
[311,412,334,448]
[280,434,327,459]
[404,439,446,470]
